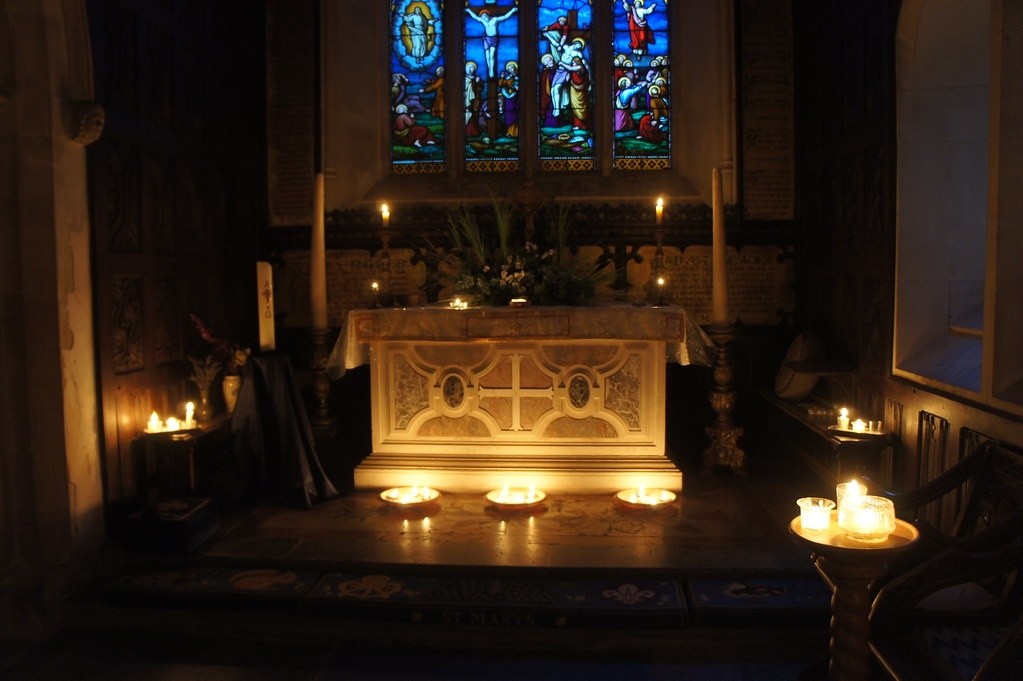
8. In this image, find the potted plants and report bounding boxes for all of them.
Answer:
[542,204,576,304]
[188,355,221,420]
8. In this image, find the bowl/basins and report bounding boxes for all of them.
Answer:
[483,487,548,511]
[613,490,679,510]
[378,486,442,509]
[838,496,896,543]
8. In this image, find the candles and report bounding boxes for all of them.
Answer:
[310,173,328,329]
[839,496,896,544]
[797,497,836,528]
[656,195,663,221]
[255,260,275,349]
[379,203,389,225]
[837,407,849,427]
[713,166,728,324]
[182,401,197,429]
[851,419,866,430]
[165,417,178,429]
[836,479,867,510]
[147,409,162,431]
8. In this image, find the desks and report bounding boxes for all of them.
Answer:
[788,516,920,681]
[133,418,229,496]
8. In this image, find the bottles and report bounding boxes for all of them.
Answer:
[223,375,241,413]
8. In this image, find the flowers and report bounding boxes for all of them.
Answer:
[421,196,540,290]
[225,346,251,375]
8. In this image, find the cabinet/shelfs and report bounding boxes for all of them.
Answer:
[773,402,897,497]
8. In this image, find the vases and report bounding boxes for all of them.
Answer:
[438,281,482,305]
[223,377,239,411]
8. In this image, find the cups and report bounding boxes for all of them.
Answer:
[835,484,868,513]
[850,422,867,433]
[869,420,881,433]
[797,497,836,532]
[837,417,849,430]
